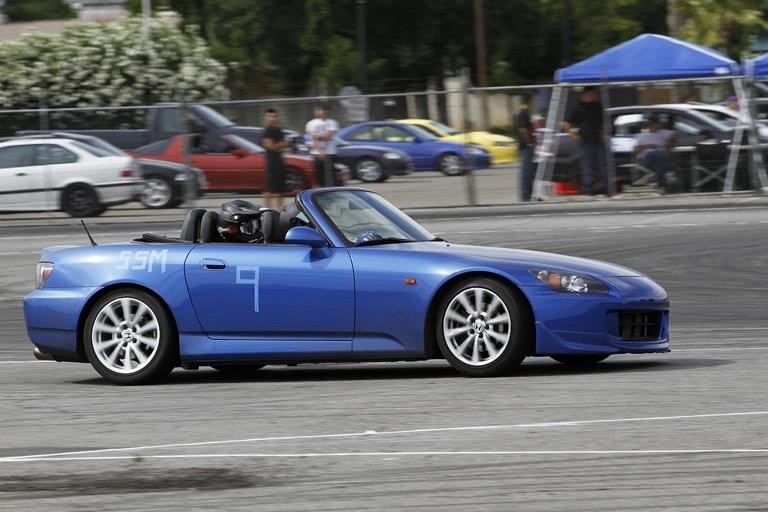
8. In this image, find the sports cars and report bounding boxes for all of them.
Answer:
[23,187,672,383]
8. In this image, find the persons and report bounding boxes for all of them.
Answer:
[560,85,612,197]
[635,115,678,187]
[513,92,544,203]
[259,108,291,211]
[305,102,339,189]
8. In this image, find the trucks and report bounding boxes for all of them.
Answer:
[16,101,297,154]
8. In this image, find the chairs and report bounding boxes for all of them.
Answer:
[261,211,289,244]
[199,210,220,243]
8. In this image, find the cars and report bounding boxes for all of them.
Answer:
[336,121,491,177]
[530,102,767,194]
[124,132,349,197]
[0,136,148,219]
[288,133,411,183]
[1,132,208,209]
[354,118,518,165]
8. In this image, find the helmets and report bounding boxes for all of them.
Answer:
[216,200,264,244]
